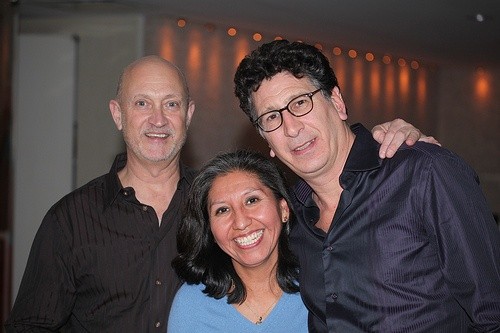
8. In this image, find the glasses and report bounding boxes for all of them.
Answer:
[252,87,323,132]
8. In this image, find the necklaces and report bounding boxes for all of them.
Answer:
[241,290,283,325]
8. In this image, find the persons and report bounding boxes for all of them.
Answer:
[6,39,500,333]
